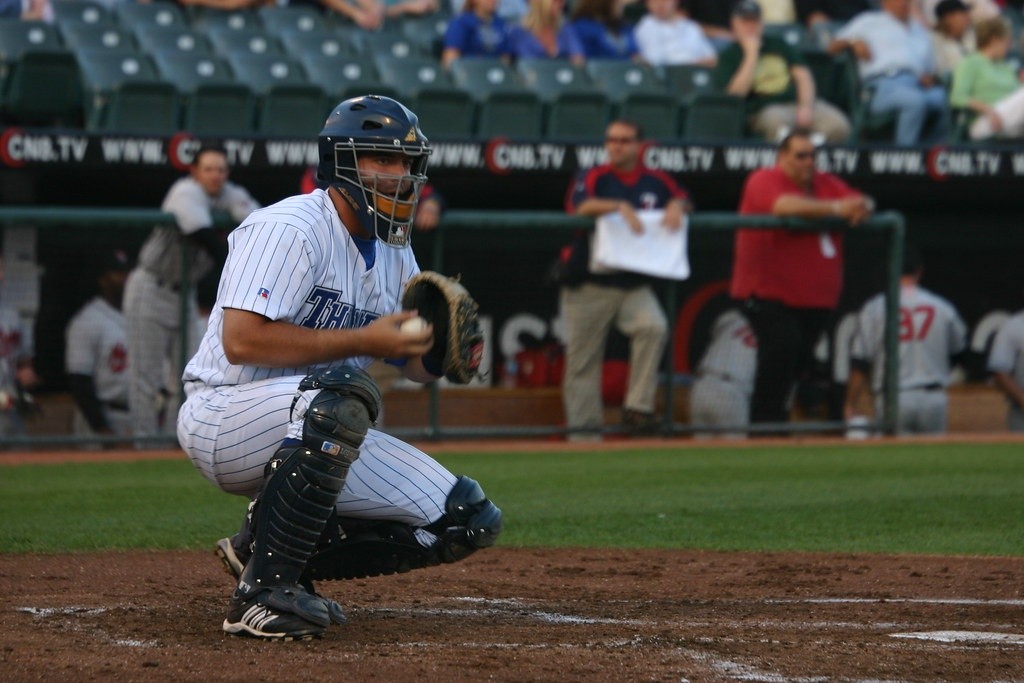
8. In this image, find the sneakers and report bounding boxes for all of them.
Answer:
[222,587,326,643]
[214,532,252,580]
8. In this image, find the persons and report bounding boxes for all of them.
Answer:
[175,93,505,642]
[730,131,872,442]
[560,116,693,443]
[1,0,1024,440]
[64,238,135,452]
[123,143,263,448]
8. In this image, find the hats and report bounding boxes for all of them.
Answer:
[733,1,760,20]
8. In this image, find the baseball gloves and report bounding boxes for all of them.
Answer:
[400,270,485,386]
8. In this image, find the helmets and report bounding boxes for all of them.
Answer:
[315,94,433,249]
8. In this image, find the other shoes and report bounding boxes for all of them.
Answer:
[623,410,657,430]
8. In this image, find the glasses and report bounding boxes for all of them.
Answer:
[789,150,818,161]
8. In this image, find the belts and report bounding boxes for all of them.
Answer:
[157,279,183,292]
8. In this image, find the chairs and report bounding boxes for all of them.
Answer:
[0,0,979,143]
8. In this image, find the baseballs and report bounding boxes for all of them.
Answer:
[400,315,430,335]
